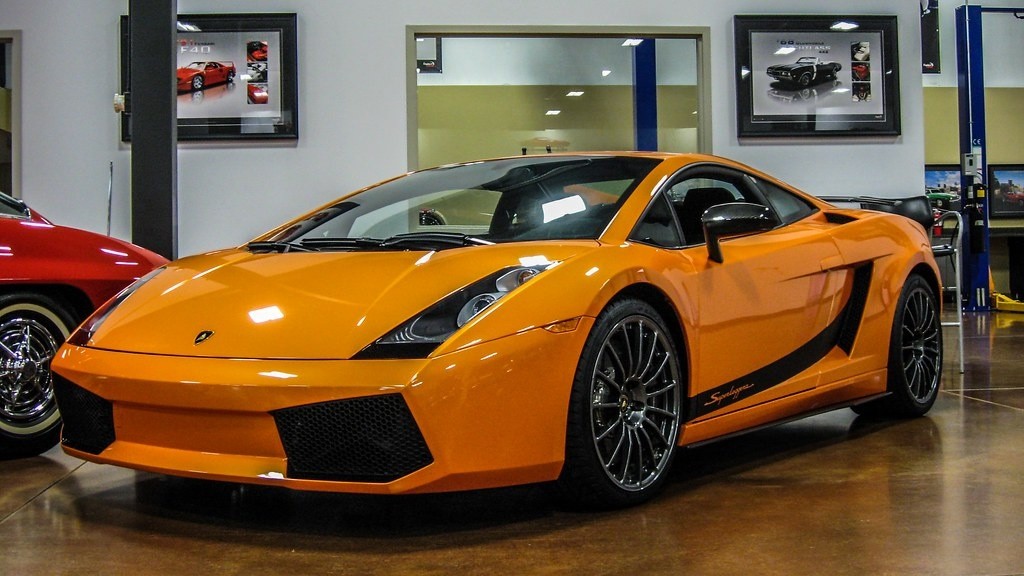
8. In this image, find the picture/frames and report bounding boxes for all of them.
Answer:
[987,163,1024,220]
[925,163,962,216]
[733,15,901,139]
[120,14,299,142]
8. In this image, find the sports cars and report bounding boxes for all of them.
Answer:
[51,154,943,508]
[766,56,842,87]
[177,59,236,92]
[1,192,173,461]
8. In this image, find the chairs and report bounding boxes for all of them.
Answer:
[615,181,685,246]
[683,188,736,245]
[860,195,963,327]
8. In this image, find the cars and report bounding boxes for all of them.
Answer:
[1001,188,1024,208]
[925,188,960,209]
